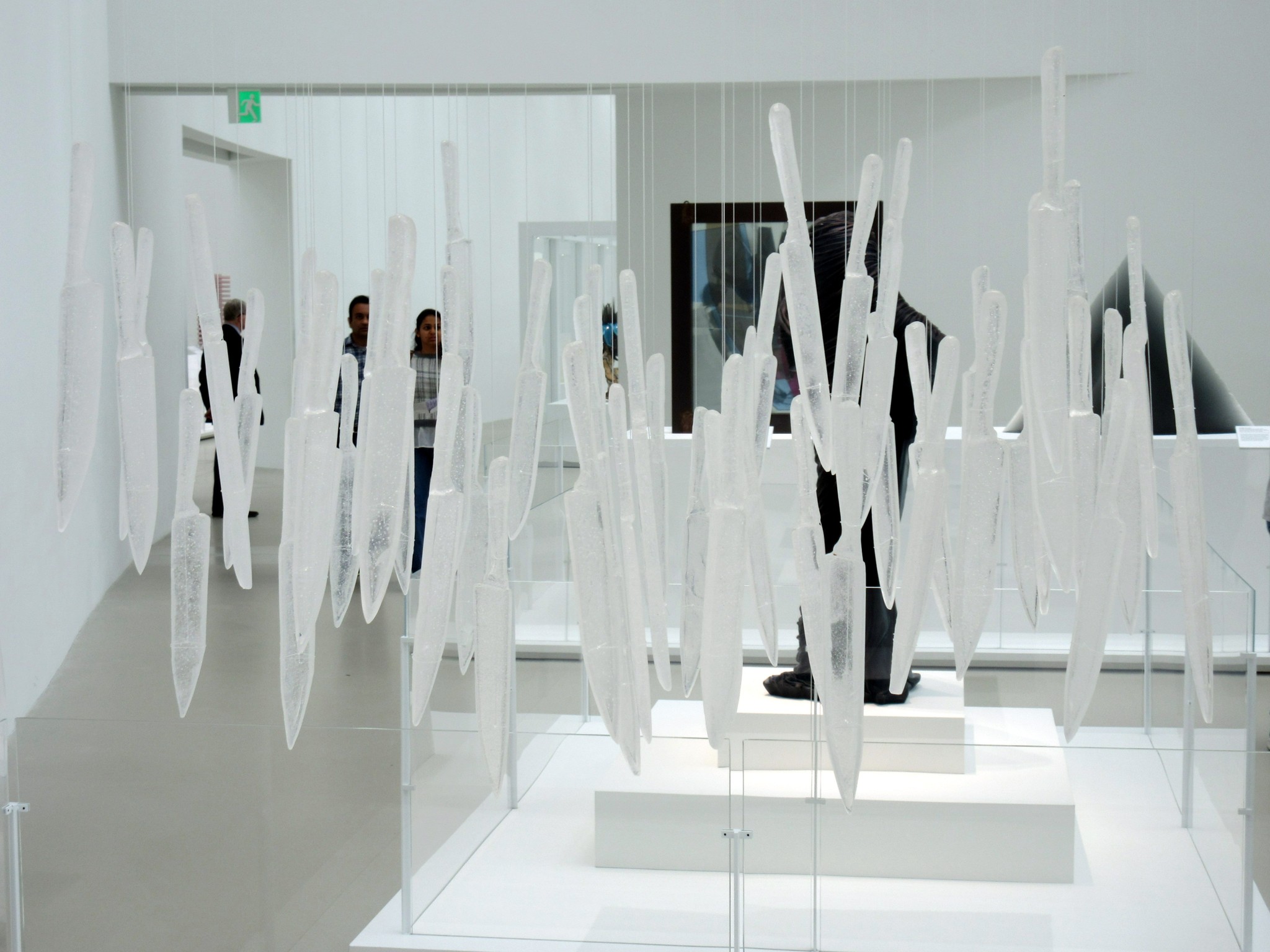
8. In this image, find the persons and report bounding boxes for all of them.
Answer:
[603,324,618,399]
[334,295,369,446]
[198,299,264,519]
[409,309,442,572]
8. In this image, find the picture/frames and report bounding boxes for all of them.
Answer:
[671,200,885,433]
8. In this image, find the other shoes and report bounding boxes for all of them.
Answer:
[211,505,259,519]
[863,674,920,706]
[765,672,823,701]
